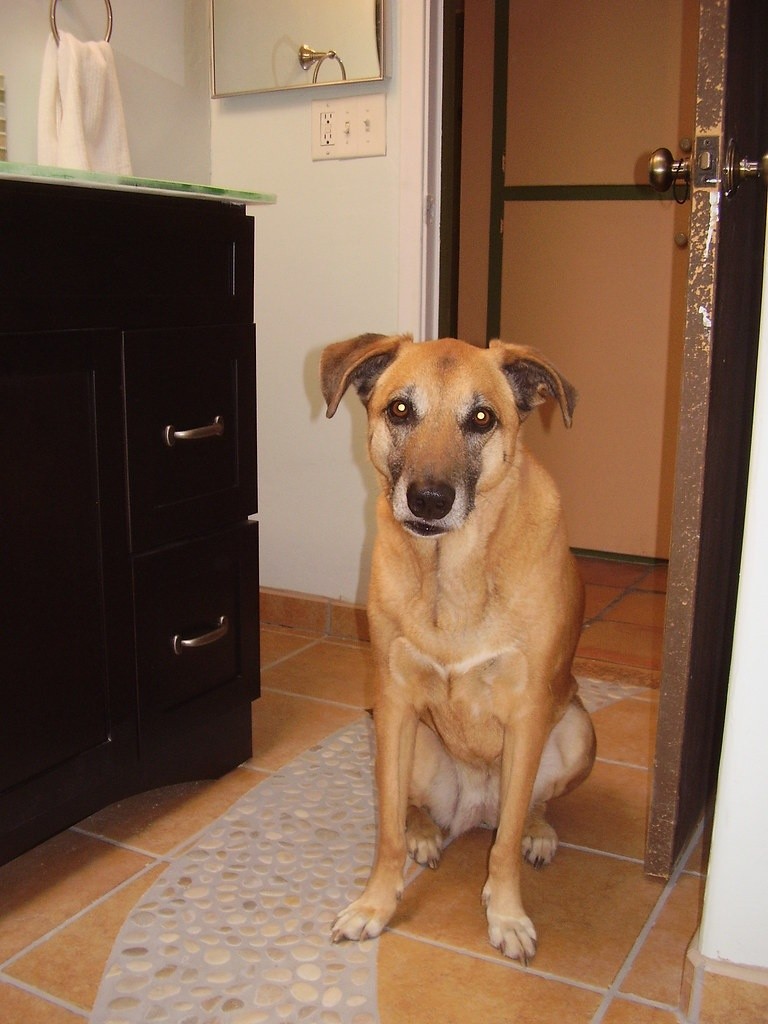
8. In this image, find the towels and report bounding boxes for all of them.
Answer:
[34,32,134,182]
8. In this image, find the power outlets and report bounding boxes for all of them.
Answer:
[310,94,387,162]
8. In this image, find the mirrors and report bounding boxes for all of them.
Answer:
[210,0,385,98]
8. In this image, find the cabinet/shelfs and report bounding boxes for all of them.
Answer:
[1,180,263,869]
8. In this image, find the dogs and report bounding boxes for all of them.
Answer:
[319,331,598,969]
